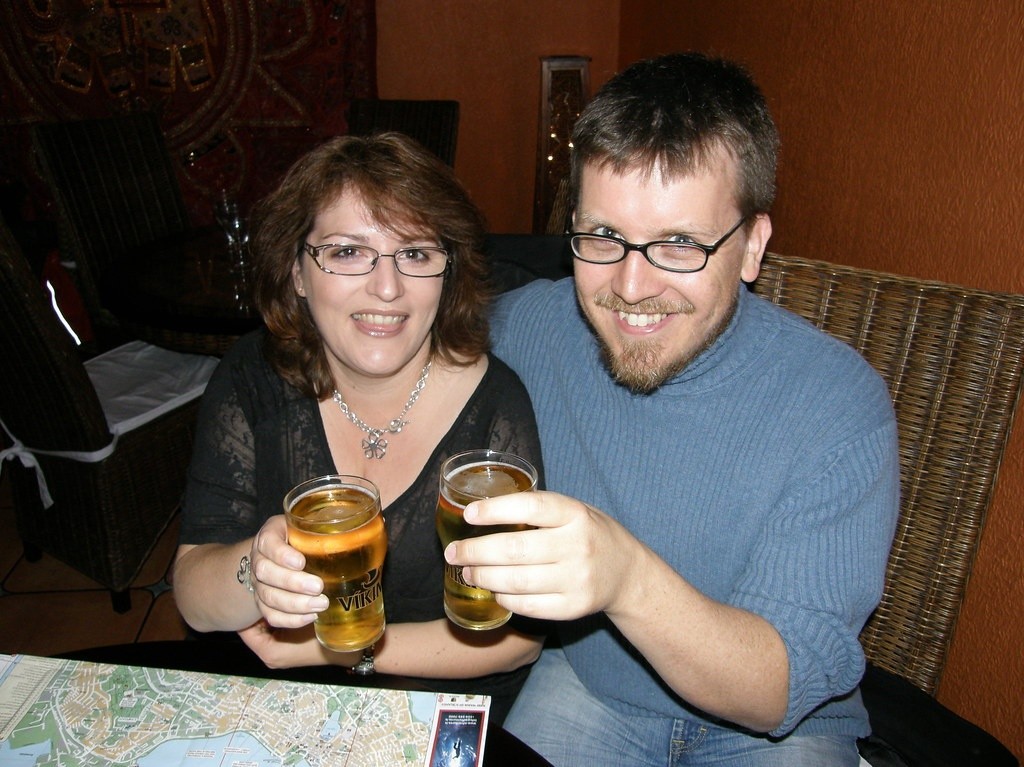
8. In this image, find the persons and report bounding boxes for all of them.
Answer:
[442,51,902,767]
[173,130,544,727]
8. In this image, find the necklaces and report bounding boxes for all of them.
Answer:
[332,361,432,459]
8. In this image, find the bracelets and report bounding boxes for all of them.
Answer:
[237,555,254,591]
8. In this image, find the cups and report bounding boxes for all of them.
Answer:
[283,475,387,653]
[435,450,539,632]
[212,191,256,273]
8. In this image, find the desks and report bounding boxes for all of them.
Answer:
[0,638,549,766]
[99,221,270,332]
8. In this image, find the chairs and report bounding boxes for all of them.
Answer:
[748,239,1021,697]
[350,95,460,168]
[30,111,245,351]
[1,216,222,618]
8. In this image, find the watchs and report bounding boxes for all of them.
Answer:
[351,643,376,676]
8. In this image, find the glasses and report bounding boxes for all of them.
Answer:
[301,238,454,278]
[563,217,748,274]
